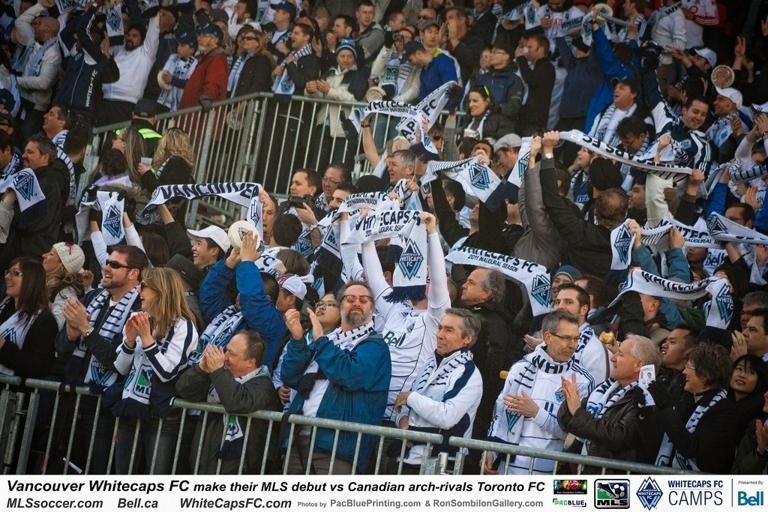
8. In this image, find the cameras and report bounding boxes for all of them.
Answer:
[289,194,315,211]
[392,33,400,41]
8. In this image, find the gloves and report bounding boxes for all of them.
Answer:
[200,93,213,112]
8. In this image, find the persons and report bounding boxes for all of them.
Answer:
[1,1,766,474]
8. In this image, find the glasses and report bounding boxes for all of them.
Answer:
[105,259,131,270]
[141,282,151,291]
[551,331,581,342]
[65,240,74,255]
[3,268,23,277]
[685,361,694,370]
[241,36,258,41]
[314,295,373,307]
[416,15,432,20]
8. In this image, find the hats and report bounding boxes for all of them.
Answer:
[134,98,156,118]
[493,133,521,153]
[270,2,296,18]
[751,103,768,113]
[610,76,637,90]
[276,274,307,300]
[715,85,742,111]
[186,225,230,254]
[53,242,85,273]
[694,48,717,67]
[335,38,360,61]
[422,21,441,32]
[178,35,198,50]
[196,23,223,41]
[399,40,422,65]
